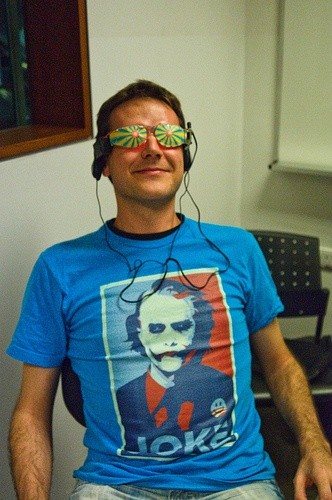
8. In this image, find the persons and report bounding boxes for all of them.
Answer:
[8,78,332,499]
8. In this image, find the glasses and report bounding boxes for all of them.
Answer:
[93,123,193,161]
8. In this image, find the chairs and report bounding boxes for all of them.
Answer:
[248,229,332,408]
